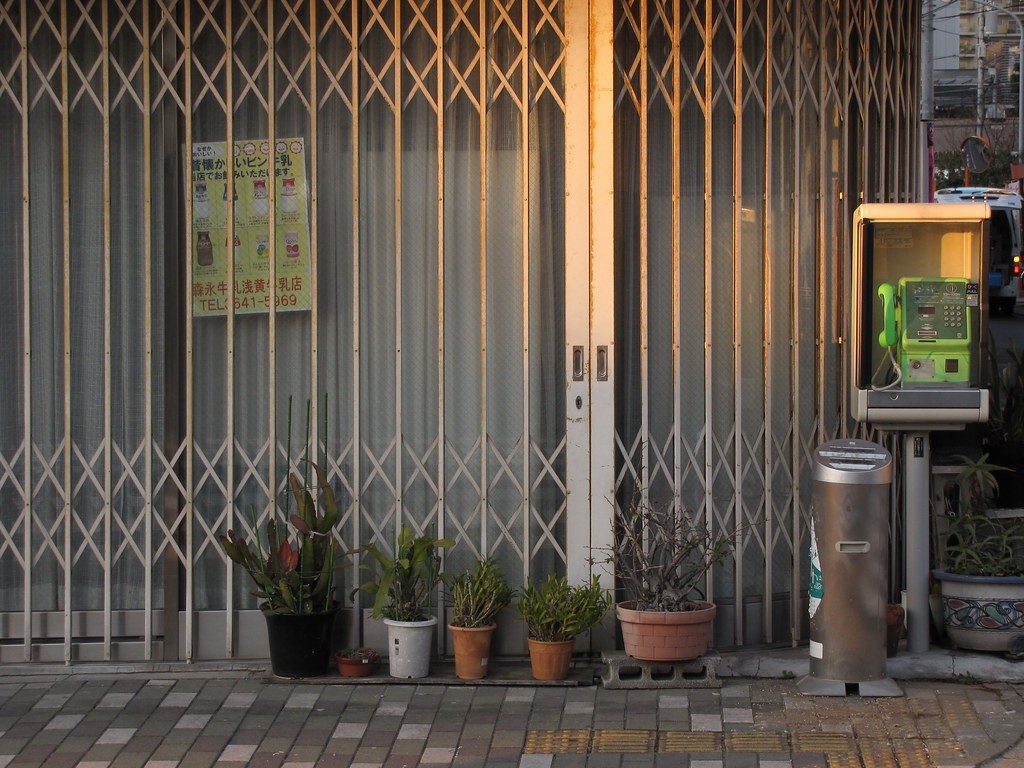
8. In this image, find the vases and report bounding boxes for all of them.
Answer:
[887,604,904,658]
[336,648,376,678]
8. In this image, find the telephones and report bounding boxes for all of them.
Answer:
[877,275,974,395]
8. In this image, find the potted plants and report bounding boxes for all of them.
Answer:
[931,455,1024,651]
[512,573,612,680]
[349,524,456,678]
[439,554,519,679]
[219,392,373,676]
[984,327,1024,510]
[581,441,771,660]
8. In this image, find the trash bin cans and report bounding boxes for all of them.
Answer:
[796,435,907,697]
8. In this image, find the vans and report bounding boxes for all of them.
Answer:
[934,186,1024,316]
